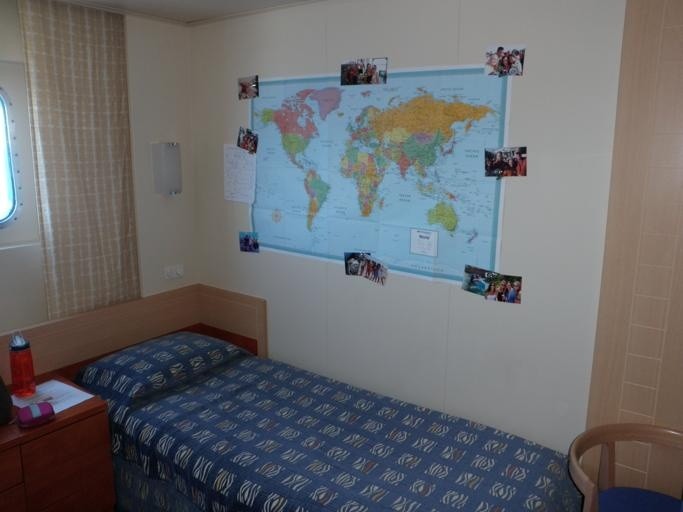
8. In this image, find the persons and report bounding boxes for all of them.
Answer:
[347,257,386,285]
[341,64,379,85]
[240,128,257,153]
[242,235,255,251]
[486,46,523,76]
[485,147,526,176]
[239,80,257,98]
[469,274,521,303]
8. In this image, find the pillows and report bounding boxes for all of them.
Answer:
[82,331,254,406]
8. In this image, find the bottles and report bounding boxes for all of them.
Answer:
[9,330,37,398]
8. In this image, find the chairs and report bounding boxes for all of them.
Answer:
[567,424,682,512]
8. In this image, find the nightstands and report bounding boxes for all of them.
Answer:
[1,372,117,511]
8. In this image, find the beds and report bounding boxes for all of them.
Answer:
[74,332,583,510]
